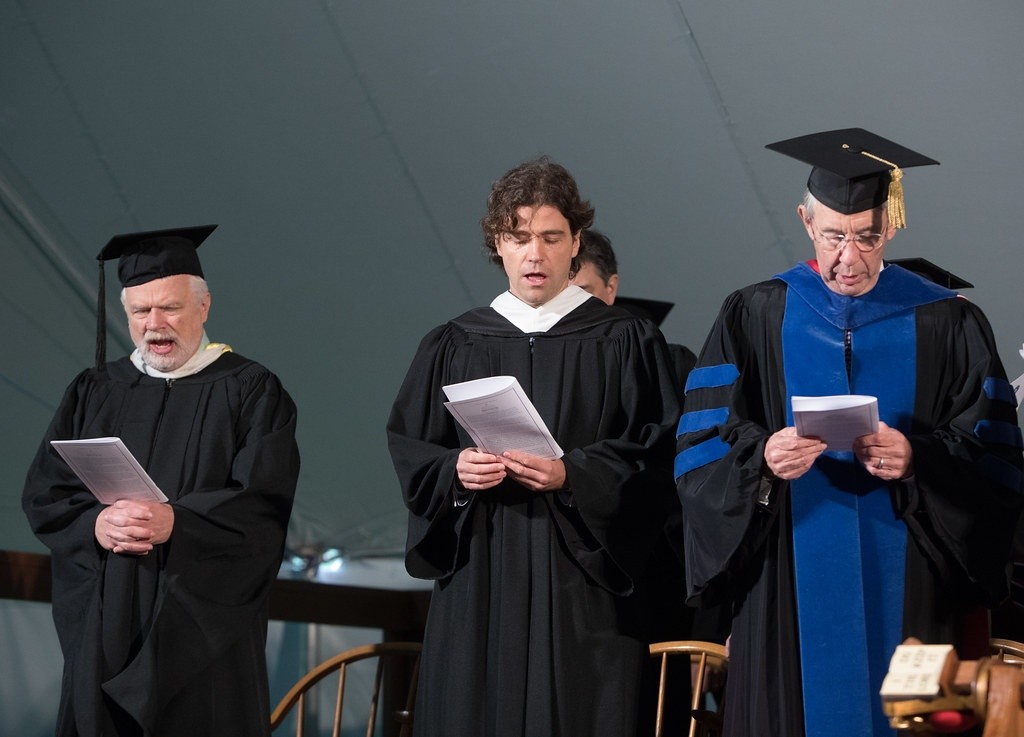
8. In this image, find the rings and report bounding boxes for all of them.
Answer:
[136,538,139,541]
[878,457,884,469]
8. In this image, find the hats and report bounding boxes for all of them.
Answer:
[765,128,941,229]
[95,225,218,369]
[883,258,974,290]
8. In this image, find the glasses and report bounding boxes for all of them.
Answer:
[809,218,883,253]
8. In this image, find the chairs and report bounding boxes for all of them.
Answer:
[647,639,728,737]
[270,642,424,737]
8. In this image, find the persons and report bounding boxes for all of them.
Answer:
[22,226,303,737]
[675,126,1024,737]
[387,155,731,737]
